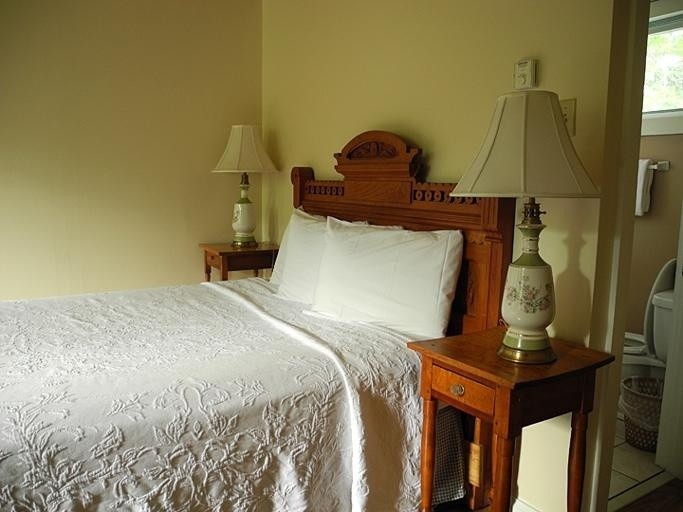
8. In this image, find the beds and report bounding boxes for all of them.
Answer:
[1,129,519,512]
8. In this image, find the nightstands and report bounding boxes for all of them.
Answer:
[198,240,282,284]
[404,325,618,512]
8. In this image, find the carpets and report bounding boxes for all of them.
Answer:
[612,475,683,512]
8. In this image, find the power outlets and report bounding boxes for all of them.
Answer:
[554,98,576,138]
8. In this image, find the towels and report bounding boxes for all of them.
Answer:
[635,157,655,218]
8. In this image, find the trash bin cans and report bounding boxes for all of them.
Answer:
[618,376,664,451]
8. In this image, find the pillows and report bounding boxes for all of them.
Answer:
[269,203,467,344]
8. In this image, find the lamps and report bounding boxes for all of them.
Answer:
[448,86,605,365]
[209,123,281,252]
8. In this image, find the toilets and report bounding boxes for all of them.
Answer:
[616,259,677,421]
